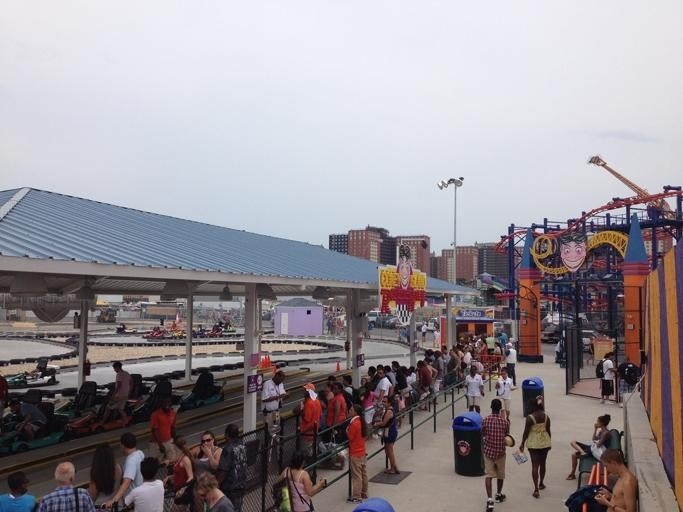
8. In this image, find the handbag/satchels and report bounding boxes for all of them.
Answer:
[382,428,389,437]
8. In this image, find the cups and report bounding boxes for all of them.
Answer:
[320,478,327,488]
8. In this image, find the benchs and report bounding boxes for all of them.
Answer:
[578,429,627,489]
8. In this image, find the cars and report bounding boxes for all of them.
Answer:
[366,310,440,332]
[535,316,616,367]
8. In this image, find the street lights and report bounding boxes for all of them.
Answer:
[437,175,465,344]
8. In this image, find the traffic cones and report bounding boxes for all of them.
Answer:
[335,360,341,372]
[259,354,276,377]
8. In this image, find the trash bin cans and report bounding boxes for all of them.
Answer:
[522,377,544,417]
[351,497,395,512]
[452,411,485,476]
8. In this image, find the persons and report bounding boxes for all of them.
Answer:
[260,370,288,464]
[8,398,48,442]
[170,321,176,330]
[420,320,426,341]
[599,352,617,404]
[295,331,517,505]
[150,397,177,460]
[594,448,638,511]
[332,318,336,334]
[1,424,249,512]
[326,318,332,334]
[116,325,228,339]
[274,451,326,511]
[101,361,133,426]
[519,395,551,499]
[336,318,341,334]
[566,414,609,480]
[1,375,9,415]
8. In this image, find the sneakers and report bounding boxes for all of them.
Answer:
[533,491,540,498]
[361,494,368,499]
[486,501,494,512]
[495,493,505,500]
[539,484,546,490]
[384,469,392,474]
[579,452,587,458]
[566,473,576,479]
[347,498,362,503]
[393,469,400,473]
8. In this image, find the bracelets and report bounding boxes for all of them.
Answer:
[612,504,618,511]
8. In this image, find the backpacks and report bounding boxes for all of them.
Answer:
[596,359,607,378]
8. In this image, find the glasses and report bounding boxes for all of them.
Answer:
[202,439,212,442]
